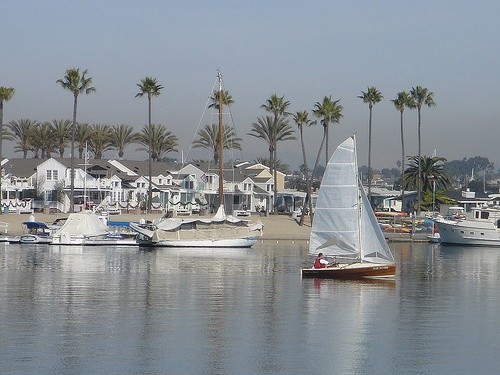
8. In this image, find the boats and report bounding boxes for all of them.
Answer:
[19,221,52,244]
[432,192,500,248]
[424,231,441,244]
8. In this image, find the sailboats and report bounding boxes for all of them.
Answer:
[299,132,397,280]
[129,65,265,249]
[49,139,123,245]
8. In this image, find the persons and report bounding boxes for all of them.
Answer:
[313,253,336,269]
